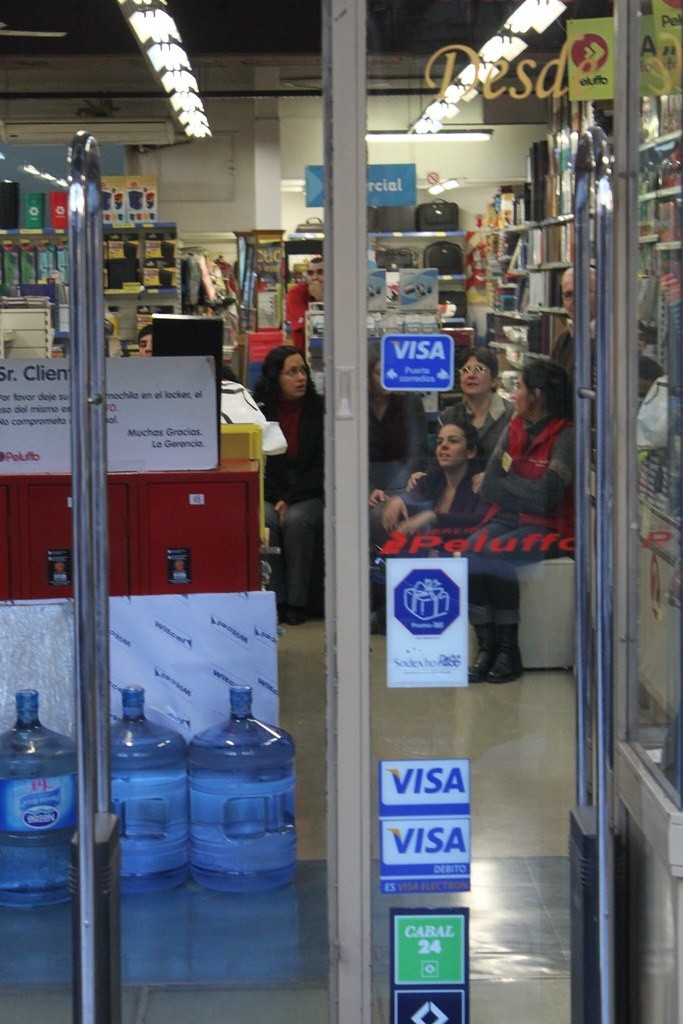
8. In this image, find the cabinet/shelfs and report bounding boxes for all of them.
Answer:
[0,224,181,361]
[297,226,468,325]
[491,128,682,391]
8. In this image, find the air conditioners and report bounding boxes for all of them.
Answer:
[0,115,174,146]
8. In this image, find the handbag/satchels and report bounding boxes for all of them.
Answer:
[438,288,468,318]
[299,197,465,276]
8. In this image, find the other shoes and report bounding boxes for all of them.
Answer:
[286,607,303,627]
[369,608,388,636]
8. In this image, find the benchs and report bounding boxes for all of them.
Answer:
[468,556,580,670]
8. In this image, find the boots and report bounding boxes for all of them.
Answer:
[487,608,523,682]
[467,618,491,683]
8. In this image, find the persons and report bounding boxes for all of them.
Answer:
[228,261,239,295]
[368,266,662,684]
[137,324,154,357]
[190,301,241,384]
[286,257,325,348]
[253,346,326,627]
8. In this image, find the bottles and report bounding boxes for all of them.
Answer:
[108,685,186,894]
[187,683,298,894]
[0,689,77,909]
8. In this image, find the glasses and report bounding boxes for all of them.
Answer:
[561,290,574,301]
[458,366,487,375]
[280,368,306,377]
